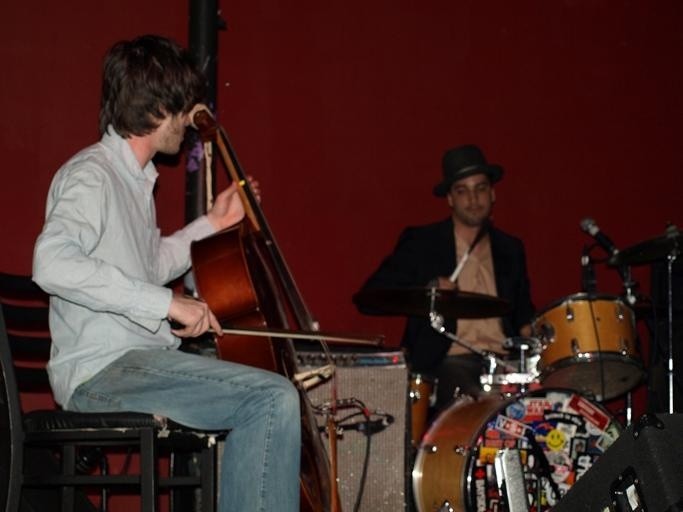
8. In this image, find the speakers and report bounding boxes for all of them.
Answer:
[297,348,411,507]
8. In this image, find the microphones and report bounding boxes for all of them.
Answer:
[429,279,441,310]
[526,429,550,469]
[574,217,622,264]
[665,222,683,262]
[185,104,219,143]
[361,420,392,437]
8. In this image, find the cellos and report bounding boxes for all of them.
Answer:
[189,102,346,512]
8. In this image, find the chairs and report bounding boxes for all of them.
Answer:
[0,311,215,507]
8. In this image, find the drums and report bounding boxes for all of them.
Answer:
[412,379,628,511]
[525,290,647,403]
[406,367,434,447]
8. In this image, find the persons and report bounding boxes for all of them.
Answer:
[26,28,302,510]
[347,134,537,428]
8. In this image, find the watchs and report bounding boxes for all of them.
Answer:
[428,276,438,290]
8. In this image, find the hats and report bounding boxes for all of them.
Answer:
[432,145,505,197]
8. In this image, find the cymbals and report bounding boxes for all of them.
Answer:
[368,287,511,320]
